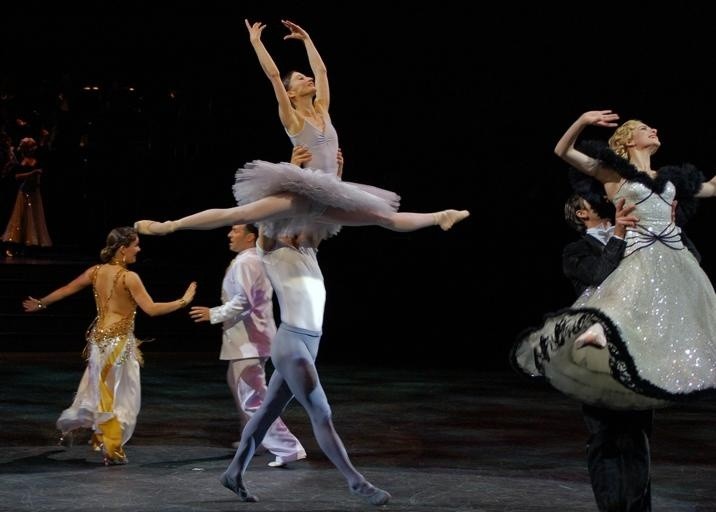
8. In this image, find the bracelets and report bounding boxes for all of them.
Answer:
[177,297,186,310]
[36,298,48,310]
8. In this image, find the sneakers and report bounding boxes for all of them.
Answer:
[574,322,608,349]
[347,480,392,507]
[218,470,260,503]
[230,434,307,468]
[133,216,175,237]
[434,207,470,231]
[91,432,131,467]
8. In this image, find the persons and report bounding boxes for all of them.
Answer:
[19,224,203,469]
[219,214,393,506]
[558,187,661,512]
[1,129,55,257]
[553,105,716,408]
[186,221,311,469]
[133,14,471,238]
